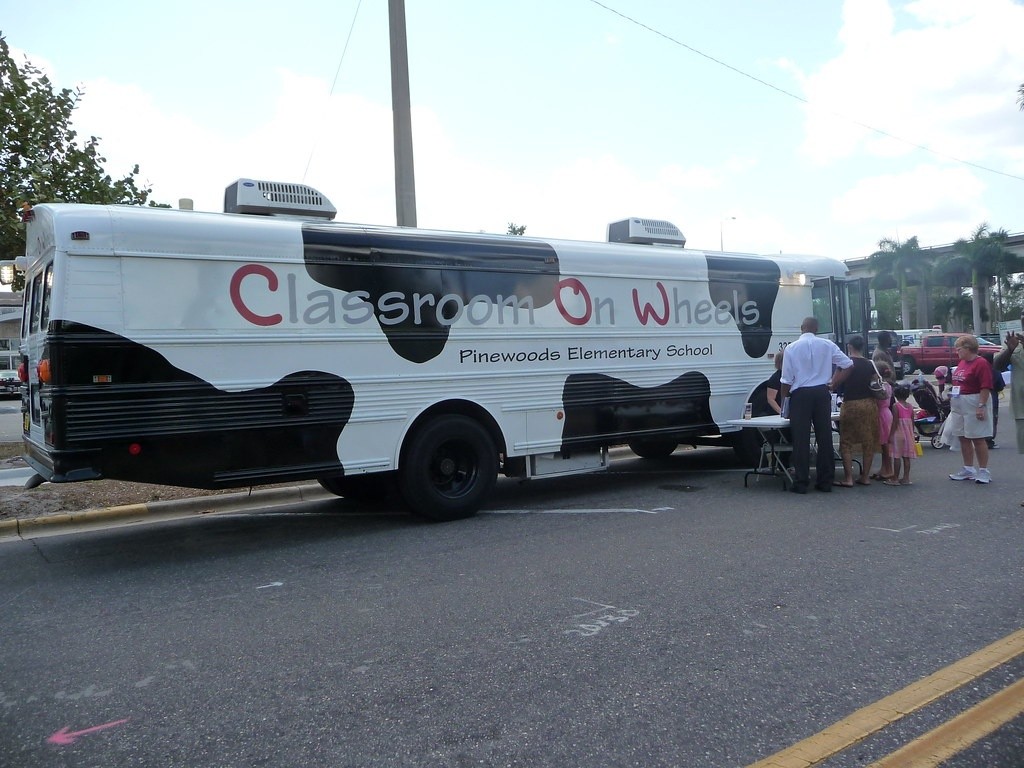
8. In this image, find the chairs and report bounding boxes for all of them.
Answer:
[756,427,794,484]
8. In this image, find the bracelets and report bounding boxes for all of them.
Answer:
[979,403,986,408]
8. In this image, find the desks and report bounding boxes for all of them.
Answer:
[726,412,862,491]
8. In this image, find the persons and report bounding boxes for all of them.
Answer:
[829,334,883,487]
[872,331,895,383]
[977,350,1006,449]
[780,317,854,494]
[949,335,994,484]
[994,330,1024,455]
[884,385,916,486]
[767,351,792,470]
[869,361,893,481]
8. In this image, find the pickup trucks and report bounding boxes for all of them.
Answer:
[901,333,1004,374]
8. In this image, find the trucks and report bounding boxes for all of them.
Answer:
[14,179,876,523]
[893,329,943,347]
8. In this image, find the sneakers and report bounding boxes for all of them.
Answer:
[975,468,992,484]
[949,466,978,480]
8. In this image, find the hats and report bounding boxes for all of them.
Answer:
[934,366,948,385]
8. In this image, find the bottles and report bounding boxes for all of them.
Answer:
[914,436,923,457]
[918,371,923,385]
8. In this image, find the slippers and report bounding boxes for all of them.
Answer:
[833,481,854,488]
[855,479,871,485]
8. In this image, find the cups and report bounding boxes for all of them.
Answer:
[745,403,752,419]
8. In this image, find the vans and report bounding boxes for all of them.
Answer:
[868,331,904,380]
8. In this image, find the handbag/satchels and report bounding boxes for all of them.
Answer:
[868,360,890,400]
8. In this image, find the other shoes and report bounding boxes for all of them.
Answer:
[815,484,831,491]
[792,487,807,494]
[883,479,900,486]
[869,473,894,480]
[899,479,913,485]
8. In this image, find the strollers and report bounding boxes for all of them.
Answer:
[910,378,951,449]
